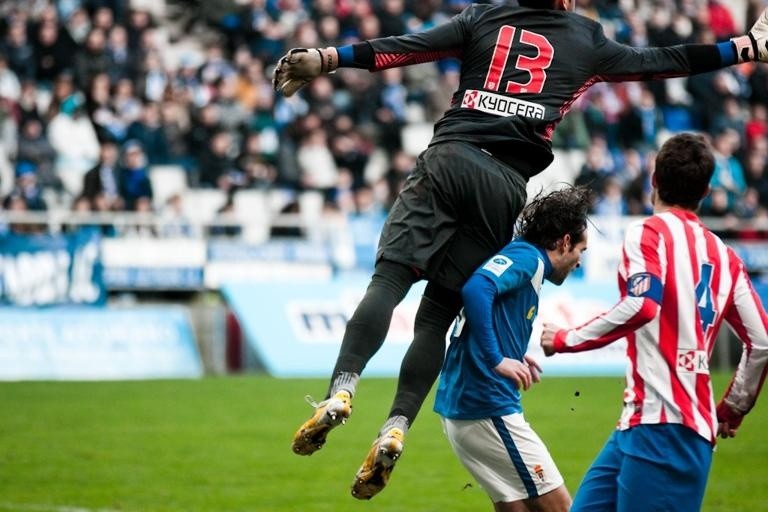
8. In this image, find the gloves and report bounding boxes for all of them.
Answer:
[730,7,768,65]
[269,44,341,100]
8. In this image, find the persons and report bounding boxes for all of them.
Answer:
[537,129,768,511]
[1,2,768,281]
[269,2,768,504]
[430,178,601,512]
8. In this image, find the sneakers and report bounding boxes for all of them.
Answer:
[349,427,405,501]
[289,390,354,457]
